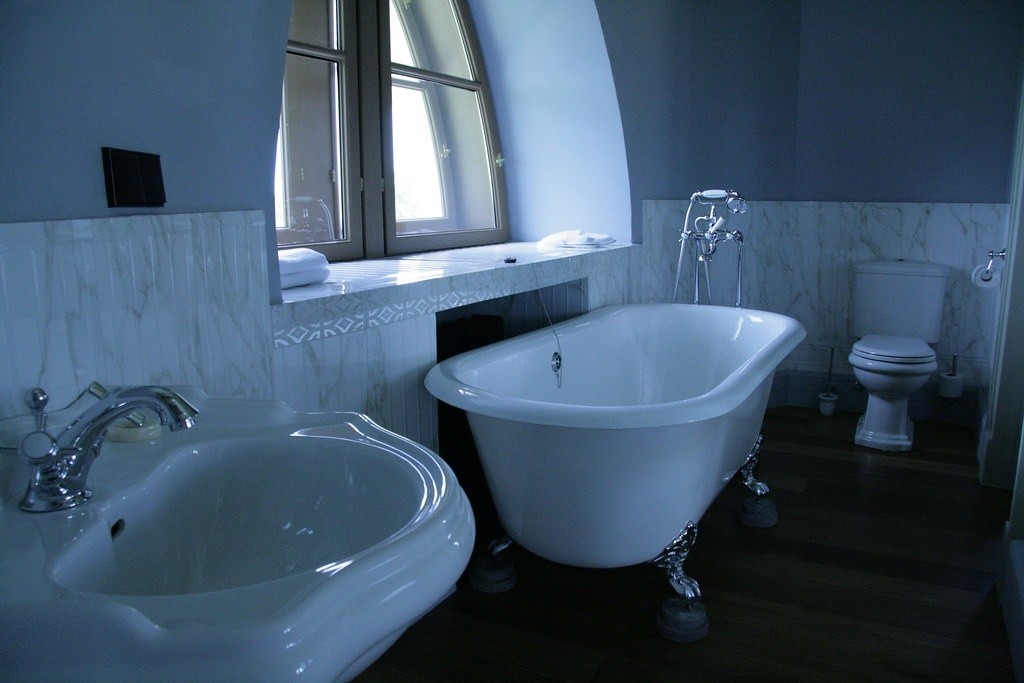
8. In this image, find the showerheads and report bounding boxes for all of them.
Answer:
[701,189,748,214]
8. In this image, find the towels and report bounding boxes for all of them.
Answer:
[276,247,329,290]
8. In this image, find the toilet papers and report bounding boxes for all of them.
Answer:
[970,262,1001,289]
[937,371,963,397]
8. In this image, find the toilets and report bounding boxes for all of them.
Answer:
[848,258,950,452]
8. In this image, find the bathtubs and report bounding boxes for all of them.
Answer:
[424,304,806,569]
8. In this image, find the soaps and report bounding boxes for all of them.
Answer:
[104,417,162,442]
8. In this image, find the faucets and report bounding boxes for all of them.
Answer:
[18,385,199,513]
[697,241,717,262]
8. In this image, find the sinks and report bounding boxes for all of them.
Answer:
[0,387,475,682]
